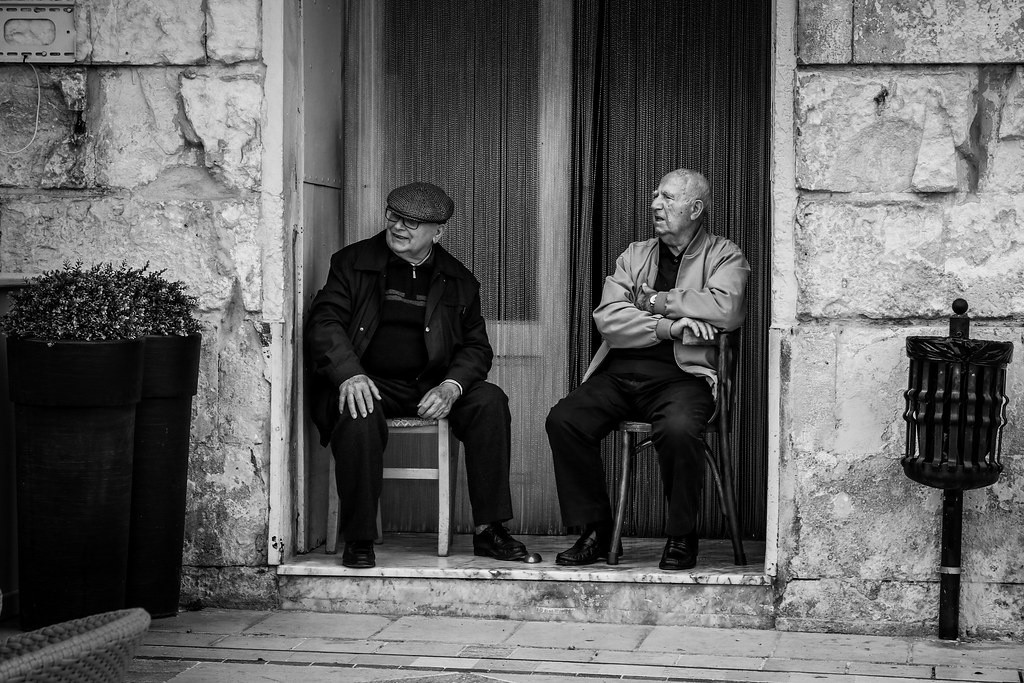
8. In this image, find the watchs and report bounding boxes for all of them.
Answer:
[650,292,658,306]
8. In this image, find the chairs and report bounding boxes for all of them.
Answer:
[324,416,459,555]
[607,326,745,566]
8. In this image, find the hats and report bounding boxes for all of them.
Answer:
[386,182,455,222]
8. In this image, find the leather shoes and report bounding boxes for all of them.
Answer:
[557,526,624,566]
[473,522,529,560]
[343,541,375,568]
[658,537,699,570]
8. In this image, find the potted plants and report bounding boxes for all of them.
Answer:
[0,253,205,631]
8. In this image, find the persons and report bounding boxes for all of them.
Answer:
[304,181,530,569]
[545,168,752,571]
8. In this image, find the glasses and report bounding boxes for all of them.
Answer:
[385,208,420,230]
